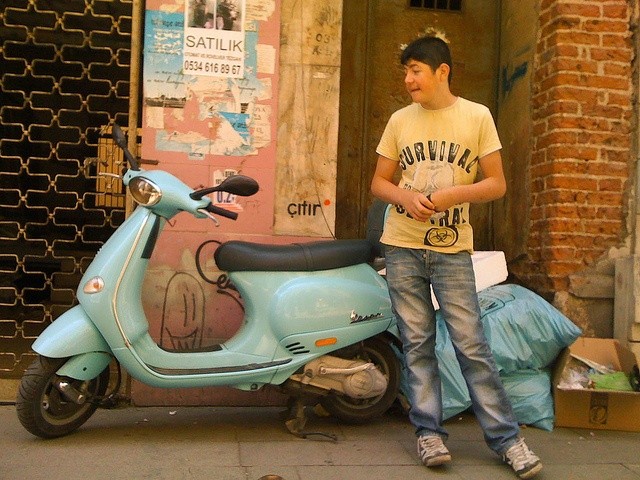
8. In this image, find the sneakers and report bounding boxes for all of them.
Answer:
[417,434,452,467]
[502,434,543,478]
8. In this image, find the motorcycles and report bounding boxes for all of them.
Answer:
[16,123,404,440]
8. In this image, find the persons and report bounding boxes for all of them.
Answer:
[370,35,545,479]
